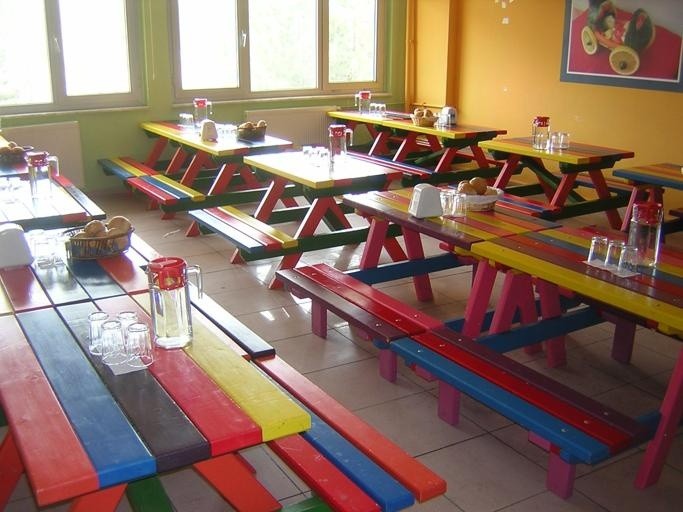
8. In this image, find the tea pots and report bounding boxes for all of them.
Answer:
[140,258,202,350]
[530,116,551,149]
[629,188,664,267]
[193,97,212,128]
[24,151,60,202]
[328,124,353,162]
[354,91,371,114]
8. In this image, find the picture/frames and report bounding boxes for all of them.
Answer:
[560,0,683,92]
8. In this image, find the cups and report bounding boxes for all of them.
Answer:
[302,144,330,167]
[586,236,638,276]
[440,189,468,218]
[29,228,68,268]
[433,112,450,128]
[0,177,23,203]
[552,131,570,149]
[370,103,386,115]
[88,312,157,368]
[177,113,196,128]
